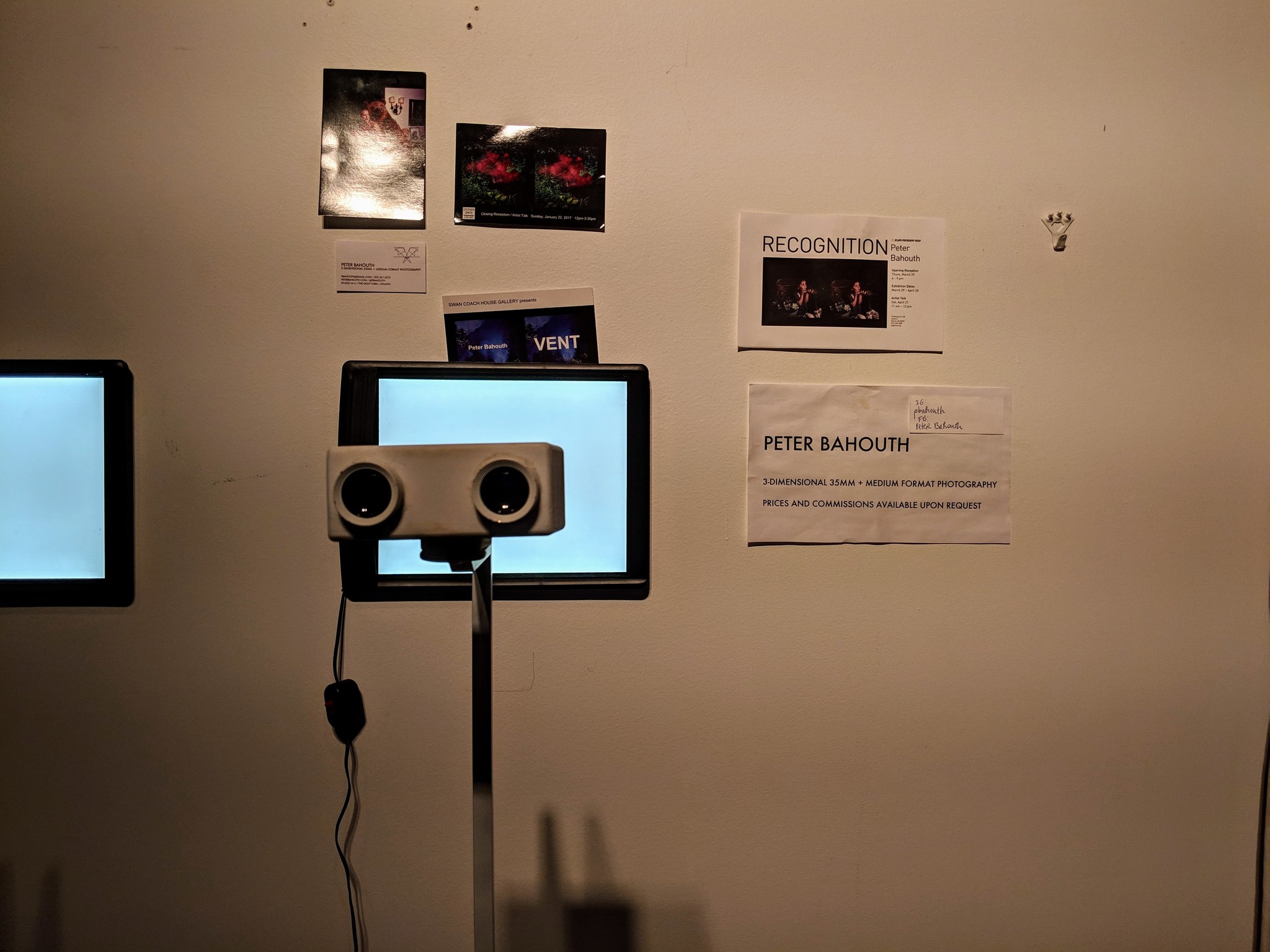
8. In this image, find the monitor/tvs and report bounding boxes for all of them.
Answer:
[335,361,651,602]
[0,358,136,608]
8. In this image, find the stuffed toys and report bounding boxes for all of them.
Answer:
[363,98,404,135]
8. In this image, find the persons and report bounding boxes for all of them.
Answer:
[845,280,868,309]
[791,278,812,310]
[359,108,382,132]
